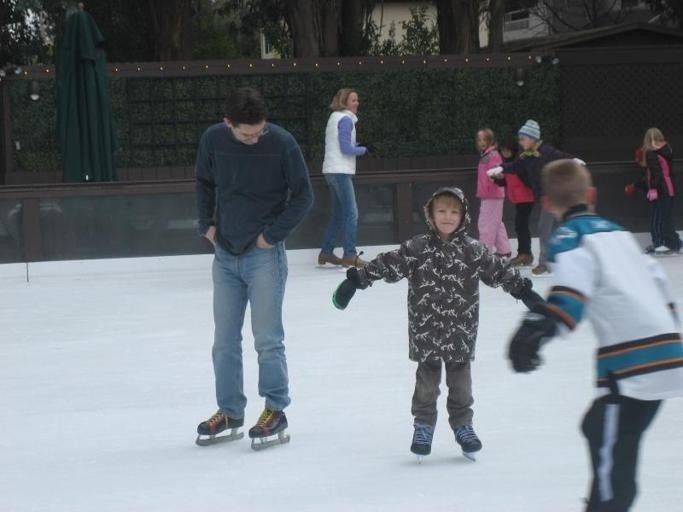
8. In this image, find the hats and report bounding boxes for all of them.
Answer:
[518,120,540,139]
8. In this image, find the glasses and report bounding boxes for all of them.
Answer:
[242,127,270,141]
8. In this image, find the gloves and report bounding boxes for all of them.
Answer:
[487,167,503,177]
[510,314,554,371]
[623,184,634,196]
[516,281,545,310]
[647,189,657,201]
[333,268,361,309]
[574,157,585,165]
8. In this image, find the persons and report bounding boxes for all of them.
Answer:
[625,128,683,255]
[509,160,683,512]
[193,88,314,439]
[332,186,543,457]
[473,127,512,258]
[317,87,370,268]
[492,130,535,267]
[486,119,586,277]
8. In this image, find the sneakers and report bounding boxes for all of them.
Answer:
[250,409,288,437]
[532,265,546,274]
[510,254,533,266]
[411,424,435,454]
[198,413,243,435]
[454,426,481,452]
[343,256,368,268]
[494,253,511,257]
[319,254,341,265]
[654,245,670,252]
